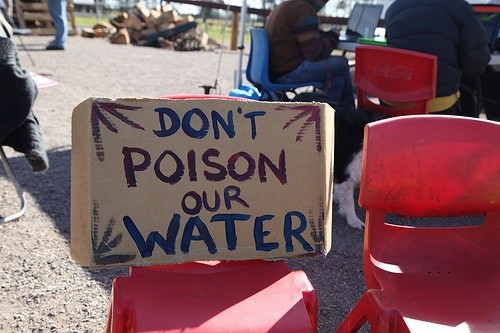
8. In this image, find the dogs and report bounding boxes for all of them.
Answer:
[291,92,386,229]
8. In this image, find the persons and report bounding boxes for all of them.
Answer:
[384,0,491,118]
[0,0,49,228]
[46,0,68,50]
[263,0,355,110]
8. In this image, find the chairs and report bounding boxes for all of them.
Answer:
[106,95,319,333]
[354,45,437,117]
[246,28,323,102]
[330,114,500,333]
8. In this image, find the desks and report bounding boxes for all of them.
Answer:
[335,31,500,68]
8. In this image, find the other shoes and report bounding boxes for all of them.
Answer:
[26,140,47,171]
[47,44,64,50]
